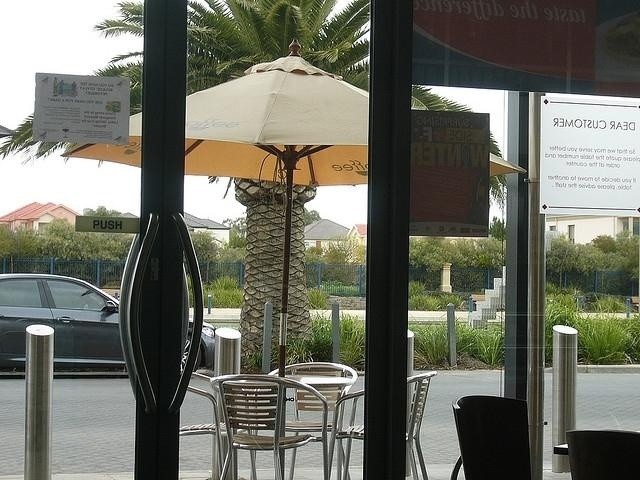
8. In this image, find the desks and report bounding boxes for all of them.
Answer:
[274,375,354,480]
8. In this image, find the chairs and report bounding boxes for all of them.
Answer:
[268,362,358,480]
[179,372,247,480]
[328,371,438,480]
[209,374,328,480]
[566,430,640,480]
[451,395,531,480]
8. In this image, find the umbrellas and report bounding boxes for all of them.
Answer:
[60,39,527,479]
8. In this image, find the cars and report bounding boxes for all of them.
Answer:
[0,273,215,372]
